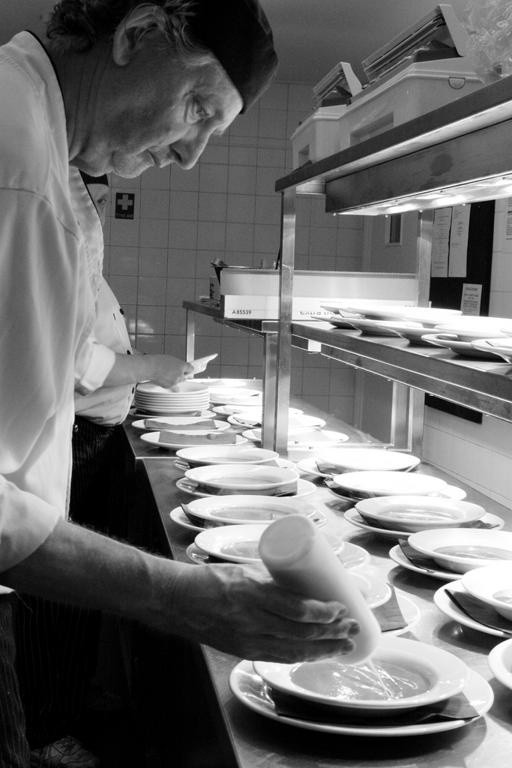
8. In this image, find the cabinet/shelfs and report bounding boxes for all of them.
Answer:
[178,76,512,426]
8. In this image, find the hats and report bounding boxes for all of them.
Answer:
[182,2,282,114]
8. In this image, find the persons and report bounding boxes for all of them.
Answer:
[70,168,196,537]
[0,0,358,768]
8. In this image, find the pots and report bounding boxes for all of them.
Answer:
[214,265,257,286]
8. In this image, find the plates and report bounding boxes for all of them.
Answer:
[312,303,512,362]
[297,448,422,476]
[176,464,316,498]
[327,471,467,502]
[343,496,504,539]
[433,564,510,639]
[129,380,349,448]
[173,448,296,469]
[387,529,510,581]
[253,637,465,709]
[168,494,329,529]
[485,640,512,691]
[229,640,493,736]
[186,523,371,571]
[242,565,417,632]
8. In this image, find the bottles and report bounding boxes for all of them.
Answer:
[259,513,382,665]
